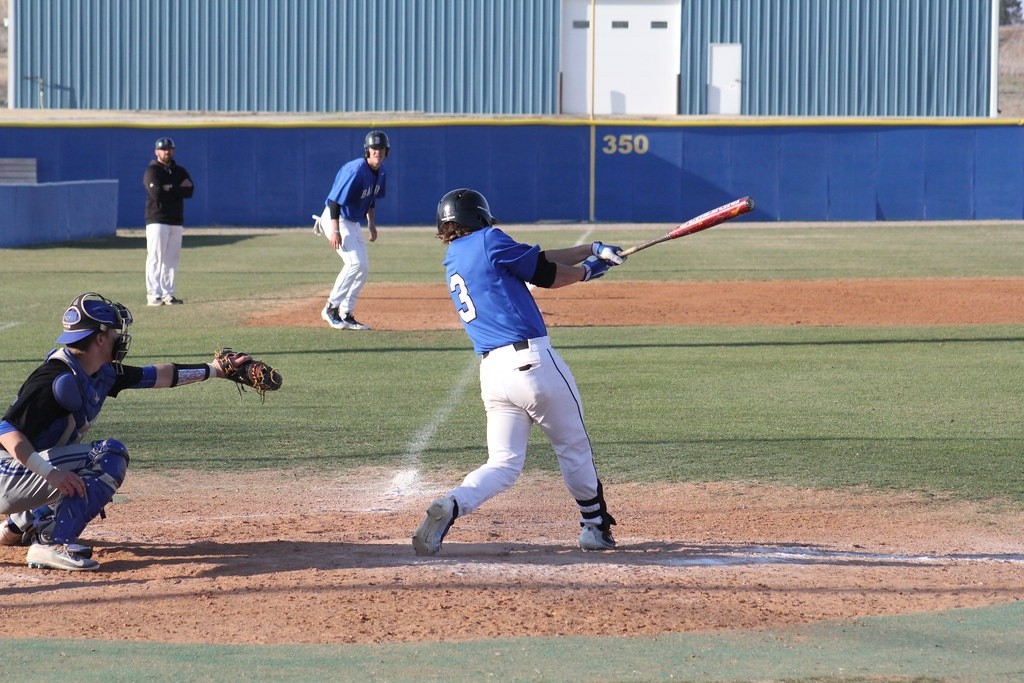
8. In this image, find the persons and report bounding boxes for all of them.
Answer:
[142,137,194,307]
[0,293,253,572]
[321,130,392,330]
[411,189,628,553]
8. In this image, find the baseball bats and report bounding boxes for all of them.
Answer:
[616,197,755,259]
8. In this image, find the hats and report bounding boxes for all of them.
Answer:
[57,300,123,343]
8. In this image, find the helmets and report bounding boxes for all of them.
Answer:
[155,138,175,149]
[436,189,496,231]
[363,130,391,157]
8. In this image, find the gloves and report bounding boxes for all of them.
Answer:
[581,255,613,281]
[311,215,323,236]
[591,242,627,266]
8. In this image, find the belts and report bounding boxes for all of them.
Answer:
[483,340,528,358]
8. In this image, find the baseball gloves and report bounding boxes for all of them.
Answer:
[214,347,282,391]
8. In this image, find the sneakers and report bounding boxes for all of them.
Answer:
[163,296,183,305]
[148,300,165,306]
[579,522,616,550]
[0,520,38,546]
[342,313,369,329]
[322,303,346,327]
[412,494,456,556]
[26,542,100,571]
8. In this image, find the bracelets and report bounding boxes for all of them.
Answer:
[334,231,340,233]
